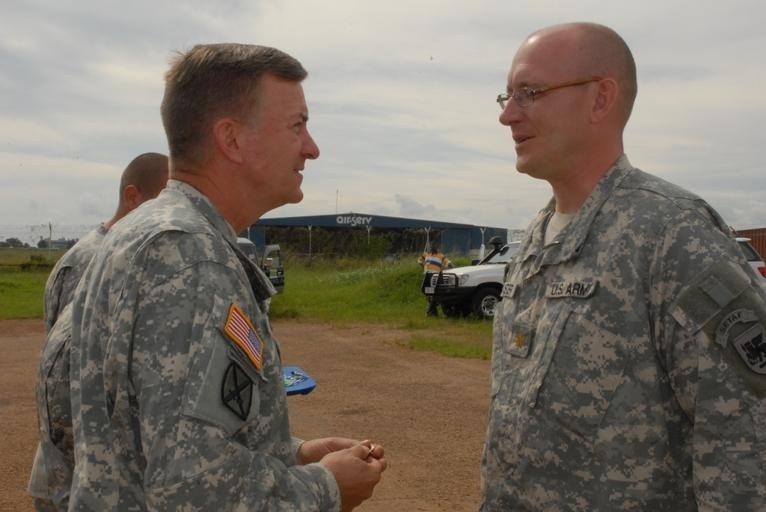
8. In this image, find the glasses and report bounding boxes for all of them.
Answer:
[495,79,600,109]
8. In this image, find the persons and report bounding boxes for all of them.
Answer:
[418,240,454,316]
[480,22,766,512]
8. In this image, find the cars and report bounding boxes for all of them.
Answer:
[235,237,284,296]
[736,237,766,294]
[421,236,521,321]
